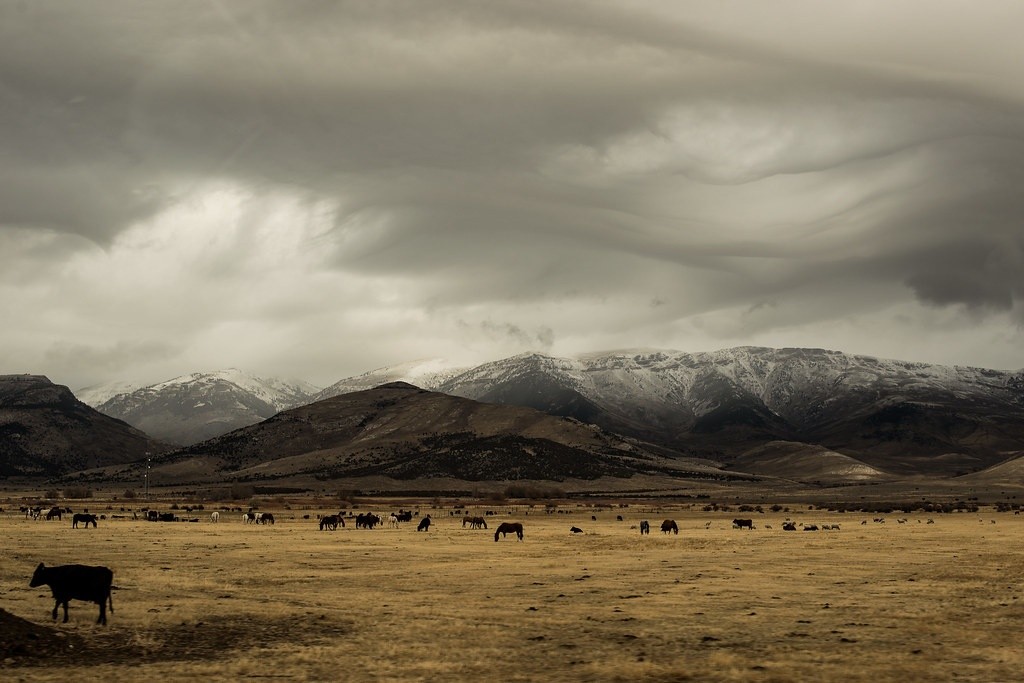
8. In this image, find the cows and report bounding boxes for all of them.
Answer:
[20,502,1019,627]
